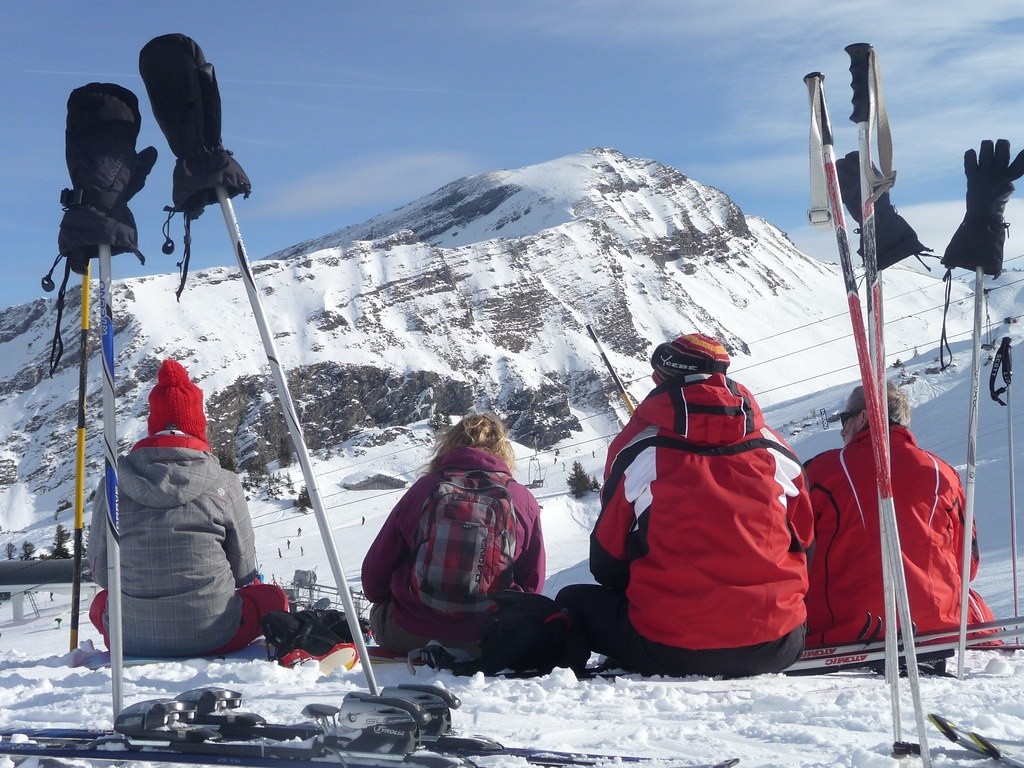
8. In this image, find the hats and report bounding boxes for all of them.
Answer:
[651,333,729,385]
[148,359,206,442]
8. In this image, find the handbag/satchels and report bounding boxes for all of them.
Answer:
[260,606,373,661]
[407,592,591,686]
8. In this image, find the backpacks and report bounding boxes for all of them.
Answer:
[401,471,522,603]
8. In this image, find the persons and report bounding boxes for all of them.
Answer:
[592,450,595,458]
[54,618,63,628]
[49,593,54,602]
[801,382,1005,648]
[361,516,365,525]
[555,332,815,680]
[562,462,566,471]
[359,410,546,658]
[554,457,557,465]
[87,359,289,658]
[276,527,305,558]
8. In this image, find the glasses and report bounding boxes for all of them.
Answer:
[840,407,861,428]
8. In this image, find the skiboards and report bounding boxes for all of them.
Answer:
[0,722,740,768]
[928,711,1021,767]
